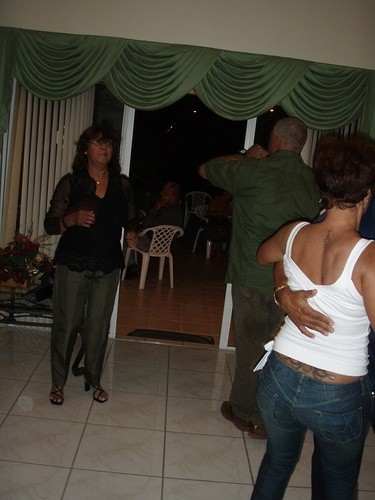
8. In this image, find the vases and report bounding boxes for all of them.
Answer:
[0,278,30,289]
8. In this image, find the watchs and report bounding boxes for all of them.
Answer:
[239,149,247,157]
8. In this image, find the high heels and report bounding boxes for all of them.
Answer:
[84,378,109,403]
[50,385,63,405]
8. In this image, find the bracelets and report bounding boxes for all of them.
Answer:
[62,216,69,228]
[273,285,286,306]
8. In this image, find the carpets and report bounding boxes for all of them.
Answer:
[128,329,214,344]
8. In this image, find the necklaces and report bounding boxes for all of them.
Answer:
[96,180,102,185]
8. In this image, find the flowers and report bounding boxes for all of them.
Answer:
[0,224,55,283]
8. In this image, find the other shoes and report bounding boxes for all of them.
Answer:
[248,424,267,440]
[221,401,251,431]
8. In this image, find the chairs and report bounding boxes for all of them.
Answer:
[184,191,212,227]
[193,205,233,257]
[122,225,184,291]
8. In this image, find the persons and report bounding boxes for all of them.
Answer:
[249,133,375,500]
[43,127,140,405]
[198,117,323,439]
[207,191,233,277]
[123,181,182,280]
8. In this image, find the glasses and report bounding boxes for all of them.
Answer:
[91,138,112,146]
